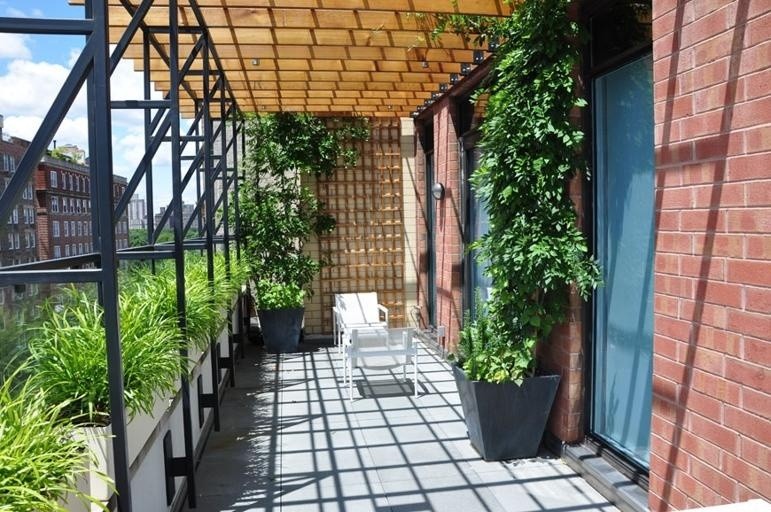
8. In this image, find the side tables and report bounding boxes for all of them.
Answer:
[344,326,389,346]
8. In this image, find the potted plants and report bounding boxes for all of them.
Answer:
[19,247,251,428]
[216,112,371,355]
[405,0,607,461]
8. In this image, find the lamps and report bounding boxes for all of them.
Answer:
[430,182,446,201]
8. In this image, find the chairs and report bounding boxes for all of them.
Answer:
[343,326,418,403]
[332,291,390,355]
